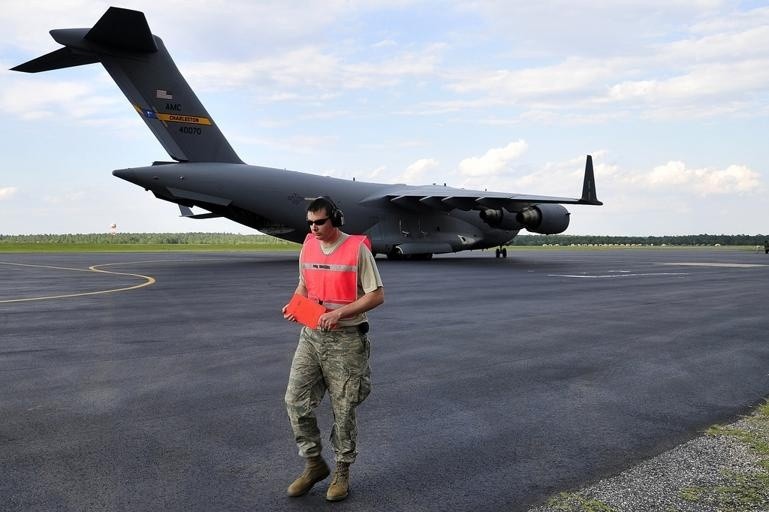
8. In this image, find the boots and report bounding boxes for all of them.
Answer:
[287,455,330,497]
[327,462,350,501]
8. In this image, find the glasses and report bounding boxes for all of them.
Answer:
[306,218,328,225]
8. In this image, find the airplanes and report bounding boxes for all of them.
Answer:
[7,6,605,261]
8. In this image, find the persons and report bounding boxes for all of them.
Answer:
[281,193,387,504]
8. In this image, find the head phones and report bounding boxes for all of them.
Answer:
[305,196,345,227]
